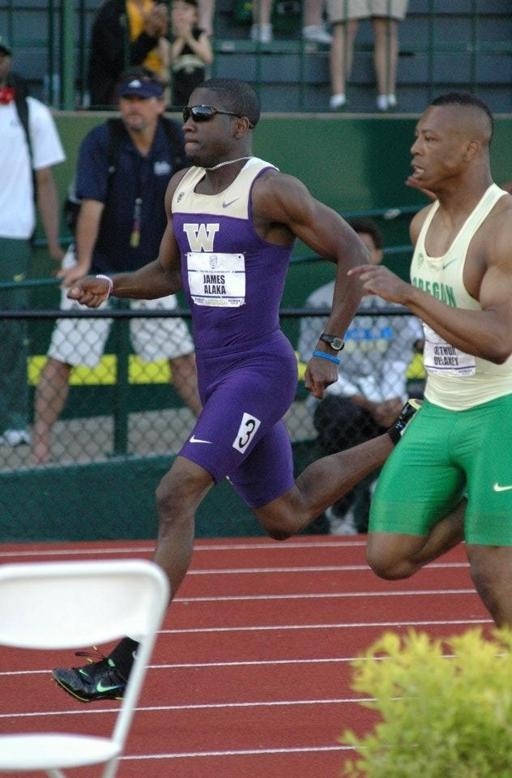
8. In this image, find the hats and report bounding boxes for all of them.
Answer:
[111,70,164,99]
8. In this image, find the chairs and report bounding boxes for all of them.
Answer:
[0,558,171,778]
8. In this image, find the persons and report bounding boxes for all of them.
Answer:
[89,0,168,108]
[24,77,204,469]
[0,37,65,446]
[345,95,511,634]
[249,0,334,45]
[326,1,406,110]
[50,77,424,703]
[299,220,425,535]
[167,2,214,108]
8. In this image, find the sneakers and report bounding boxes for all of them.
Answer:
[393,397,421,437]
[326,506,355,535]
[51,651,130,703]
[4,428,31,447]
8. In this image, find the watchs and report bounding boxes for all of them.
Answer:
[320,333,345,352]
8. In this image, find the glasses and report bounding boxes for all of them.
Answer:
[183,104,255,129]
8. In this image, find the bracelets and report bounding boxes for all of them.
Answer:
[96,273,114,302]
